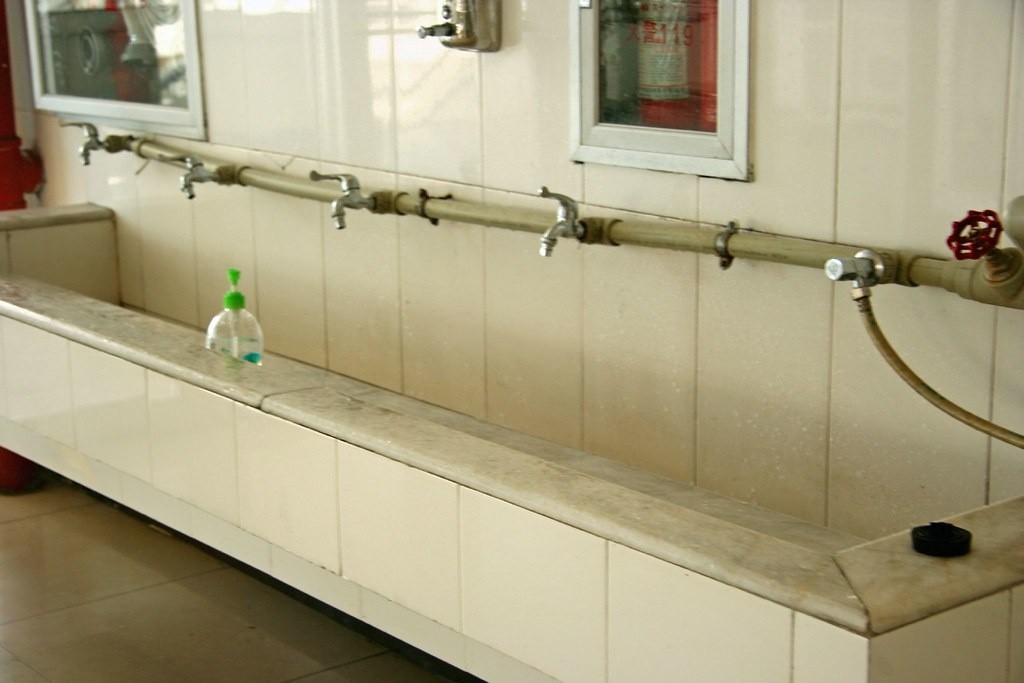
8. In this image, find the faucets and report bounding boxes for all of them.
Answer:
[57,122,109,166]
[157,151,221,200]
[537,185,585,258]
[307,170,377,231]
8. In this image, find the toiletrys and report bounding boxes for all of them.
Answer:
[203,267,265,370]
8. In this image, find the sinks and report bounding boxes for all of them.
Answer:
[1,201,1024,683]
